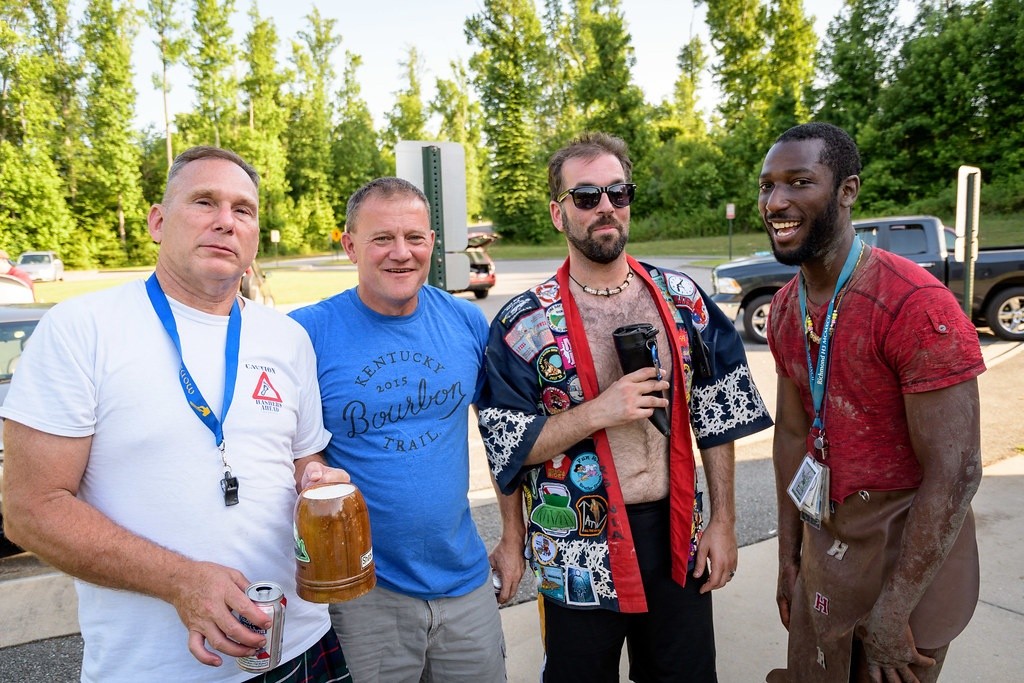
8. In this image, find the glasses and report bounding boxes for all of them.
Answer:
[557,183,637,209]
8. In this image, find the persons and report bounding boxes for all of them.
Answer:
[0,147,354,683]
[758,121,988,683]
[474,130,775,683]
[285,177,527,683]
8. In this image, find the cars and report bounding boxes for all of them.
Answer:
[17,252,64,282]
[0,252,35,303]
[0,302,54,556]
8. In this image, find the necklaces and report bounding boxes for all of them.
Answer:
[568,261,635,297]
[804,239,865,344]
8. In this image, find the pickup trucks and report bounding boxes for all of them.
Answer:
[706,215,1024,345]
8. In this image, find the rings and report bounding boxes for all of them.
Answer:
[729,569,735,577]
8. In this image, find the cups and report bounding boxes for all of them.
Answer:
[293,482,376,603]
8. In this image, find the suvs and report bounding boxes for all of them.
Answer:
[449,247,495,299]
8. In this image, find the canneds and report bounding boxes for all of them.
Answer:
[235,581,287,673]
